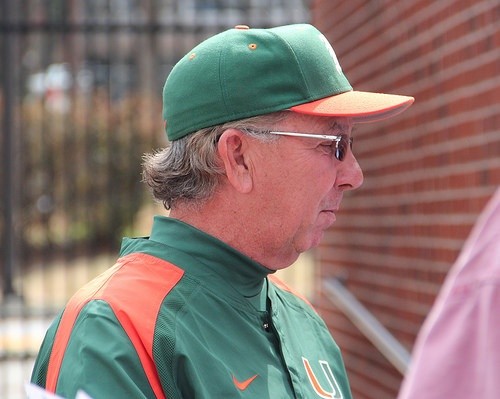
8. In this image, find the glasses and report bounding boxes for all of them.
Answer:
[213,127,353,162]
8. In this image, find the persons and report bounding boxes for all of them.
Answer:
[30,23,416,399]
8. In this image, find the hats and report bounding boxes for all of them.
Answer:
[162,24,414,142]
